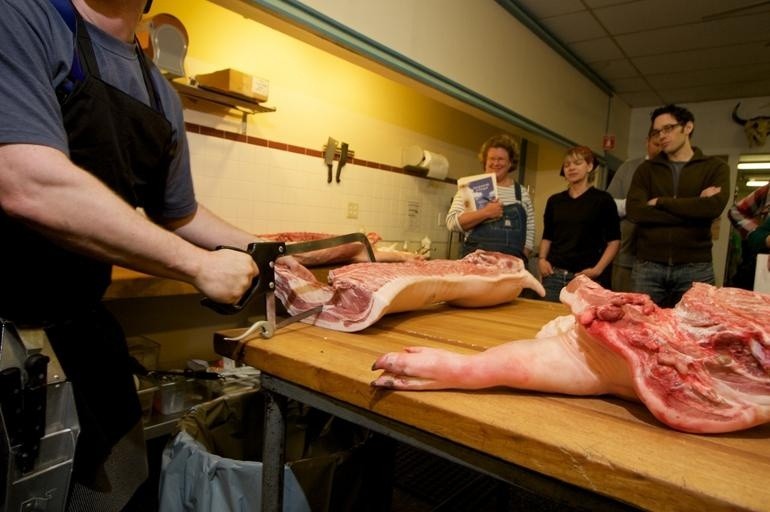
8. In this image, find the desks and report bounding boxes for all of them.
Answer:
[213,295,770,512]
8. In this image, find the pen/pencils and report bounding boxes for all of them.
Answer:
[482,197,491,202]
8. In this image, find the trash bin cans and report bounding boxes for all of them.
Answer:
[160,389,394,512]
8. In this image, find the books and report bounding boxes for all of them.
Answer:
[457,173,499,224]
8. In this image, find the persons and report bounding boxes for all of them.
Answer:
[445,134,535,270]
[0,1,316,512]
[727,181,770,292]
[624,105,733,310]
[606,126,663,293]
[536,145,621,304]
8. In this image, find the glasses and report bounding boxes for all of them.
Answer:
[488,157,508,165]
[650,122,679,136]
